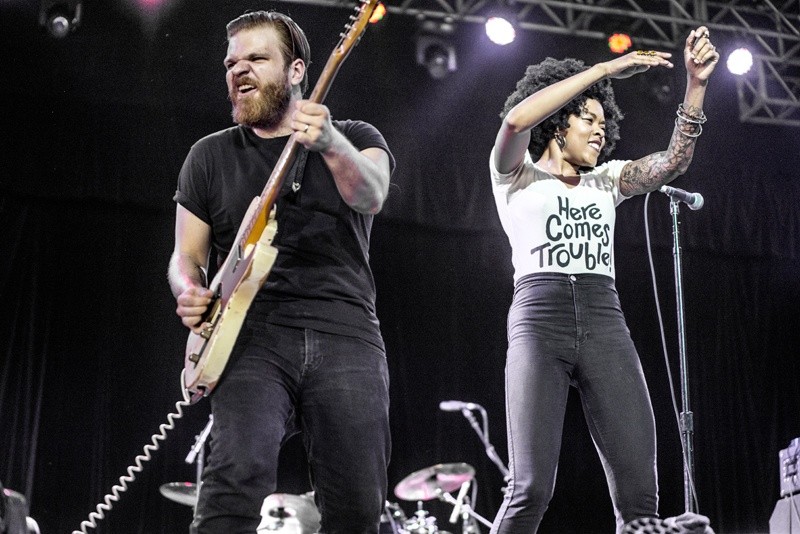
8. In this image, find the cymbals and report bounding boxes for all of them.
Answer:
[159,482,204,505]
[257,492,321,534]
[394,462,476,501]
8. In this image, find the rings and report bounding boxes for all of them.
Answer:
[303,124,309,133]
[637,50,657,56]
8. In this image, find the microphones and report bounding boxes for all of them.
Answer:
[440,401,481,410]
[659,184,705,210]
[449,480,471,523]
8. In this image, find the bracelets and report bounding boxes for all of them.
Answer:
[674,103,707,138]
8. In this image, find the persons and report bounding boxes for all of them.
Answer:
[165,9,397,534]
[487,25,720,534]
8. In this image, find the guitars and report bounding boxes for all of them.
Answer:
[182,0,378,397]
